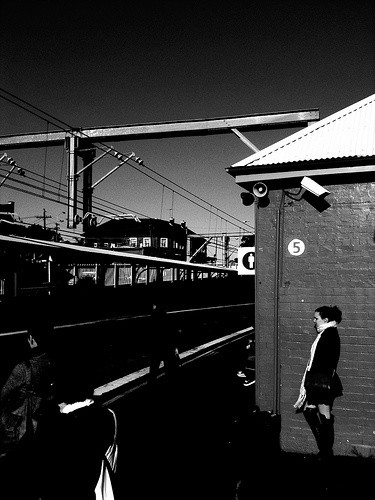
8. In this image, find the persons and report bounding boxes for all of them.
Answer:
[293,305,345,473]
[148,296,181,395]
[11,358,122,500]
[0,313,66,478]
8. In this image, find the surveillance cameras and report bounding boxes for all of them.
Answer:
[301,178,330,199]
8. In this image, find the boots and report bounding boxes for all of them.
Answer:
[318,411,335,450]
[302,408,332,456]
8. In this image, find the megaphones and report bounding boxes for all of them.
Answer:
[251,182,269,198]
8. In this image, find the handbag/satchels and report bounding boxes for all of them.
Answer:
[0,384,37,447]
